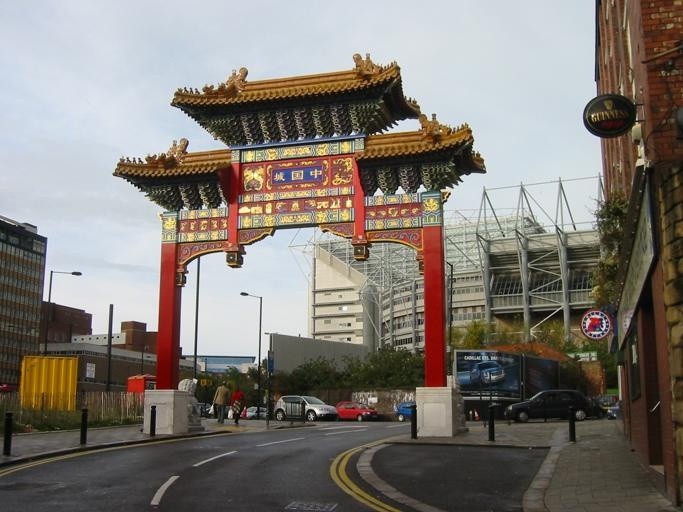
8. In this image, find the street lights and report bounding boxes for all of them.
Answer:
[141,344,150,374]
[240,292,263,418]
[44,270,82,357]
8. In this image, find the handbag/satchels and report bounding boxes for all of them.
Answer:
[209,405,216,415]
[227,409,235,420]
[239,407,249,418]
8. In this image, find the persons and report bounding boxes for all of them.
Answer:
[227,382,245,424]
[212,379,231,423]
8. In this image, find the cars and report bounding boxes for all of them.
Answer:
[393,401,417,422]
[336,401,379,422]
[198,402,266,420]
[471,360,505,384]
[503,389,599,423]
[273,395,338,422]
